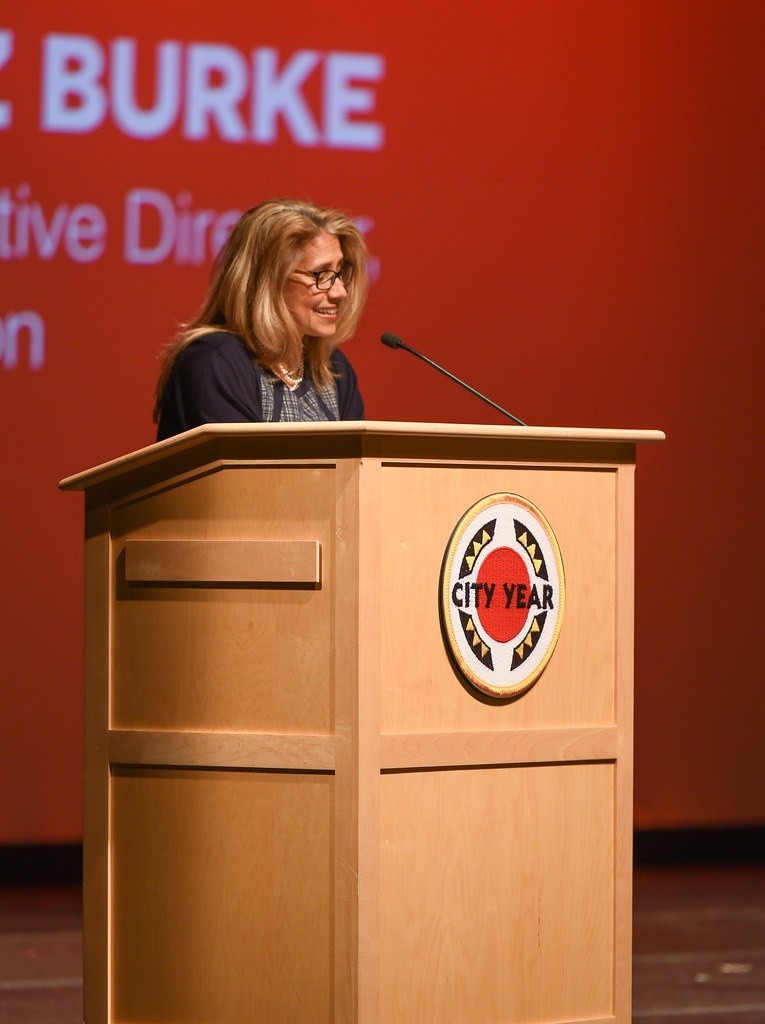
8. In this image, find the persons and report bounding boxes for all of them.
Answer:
[152,199,370,444]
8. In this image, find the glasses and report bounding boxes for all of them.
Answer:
[291,262,356,290]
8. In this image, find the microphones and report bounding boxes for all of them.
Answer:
[381,333,527,428]
